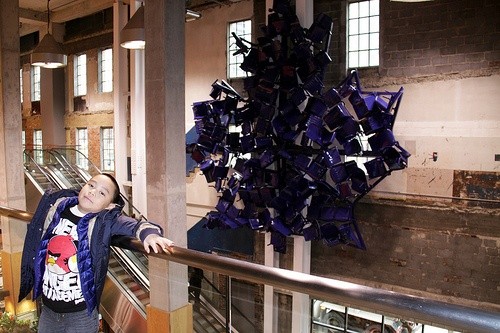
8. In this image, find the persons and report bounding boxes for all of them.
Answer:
[17,172,175,333]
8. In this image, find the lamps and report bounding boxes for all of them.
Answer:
[30,0,68,69]
